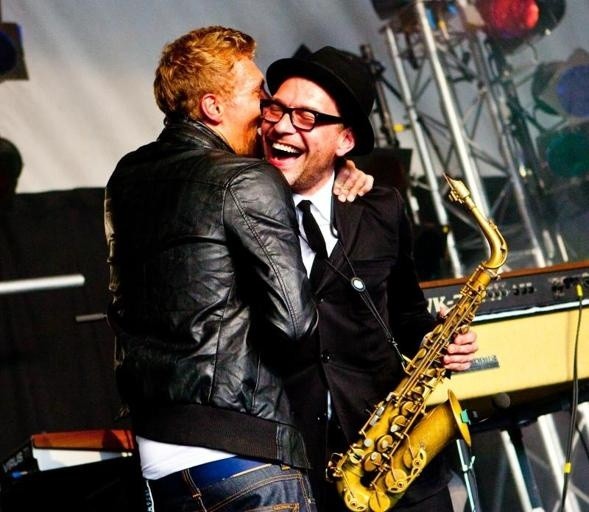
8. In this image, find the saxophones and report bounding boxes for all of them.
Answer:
[325,175,509,512]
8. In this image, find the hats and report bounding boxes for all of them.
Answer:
[265,45,375,155]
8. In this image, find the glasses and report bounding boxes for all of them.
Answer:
[258,98,344,131]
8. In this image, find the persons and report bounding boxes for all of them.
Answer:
[253,44,480,512]
[102,24,378,510]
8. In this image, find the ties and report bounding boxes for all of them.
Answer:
[296,201,328,287]
